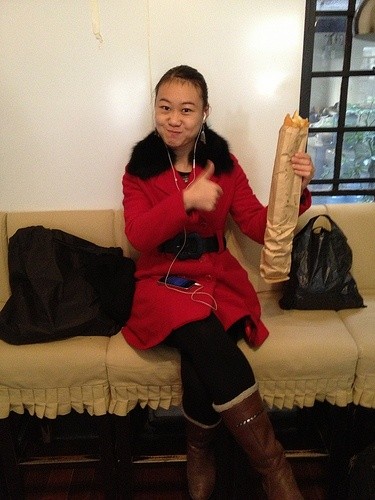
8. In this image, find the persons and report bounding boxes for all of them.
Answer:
[124,64,314,500]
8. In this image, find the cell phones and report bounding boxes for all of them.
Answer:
[157,276,196,290]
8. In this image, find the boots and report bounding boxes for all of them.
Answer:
[183,414,220,500]
[212,383,304,500]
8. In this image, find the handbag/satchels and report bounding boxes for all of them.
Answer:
[279,215,365,310]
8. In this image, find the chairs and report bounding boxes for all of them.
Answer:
[0,203,375,467]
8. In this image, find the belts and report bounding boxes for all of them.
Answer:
[157,233,228,258]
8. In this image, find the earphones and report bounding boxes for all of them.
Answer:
[202,112,207,123]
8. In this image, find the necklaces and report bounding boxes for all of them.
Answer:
[181,162,190,182]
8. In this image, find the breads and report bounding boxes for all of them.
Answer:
[283,110,308,128]
